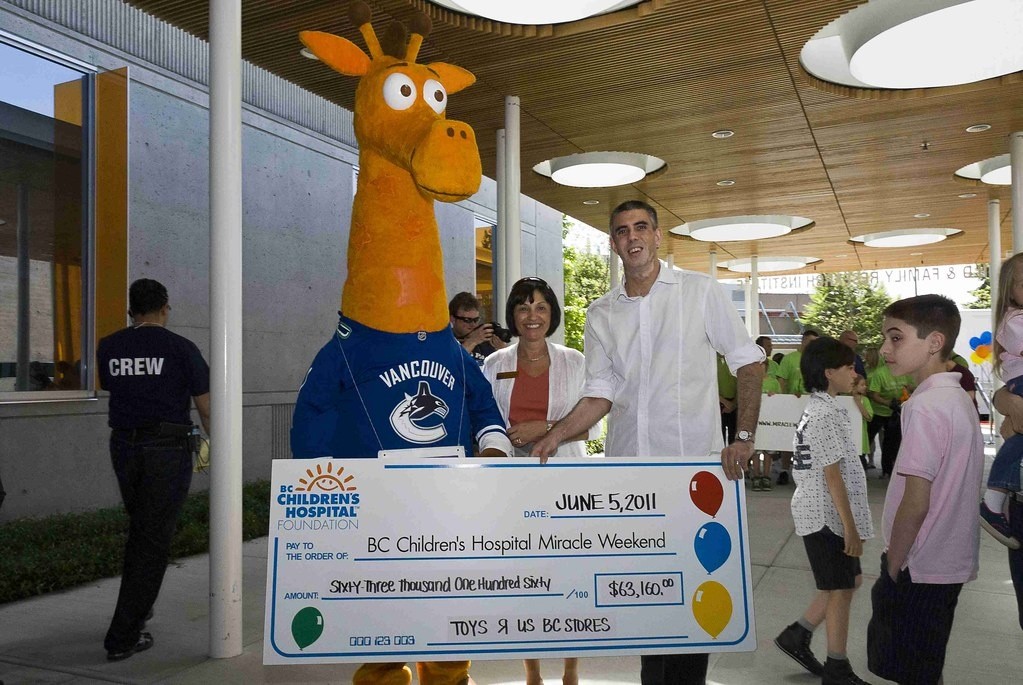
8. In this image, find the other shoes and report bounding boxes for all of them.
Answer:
[750,476,761,490]
[775,471,789,484]
[761,476,772,491]
[868,460,876,469]
[106,632,153,660]
[878,471,888,479]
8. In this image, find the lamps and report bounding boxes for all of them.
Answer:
[979,152,1013,186]
[839,0,1023,89]
[864,227,948,248]
[726,256,807,272]
[688,215,793,242]
[550,151,647,188]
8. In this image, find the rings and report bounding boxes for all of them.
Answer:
[734,460,741,464]
[517,438,522,443]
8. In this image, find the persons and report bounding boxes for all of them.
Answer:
[529,200,766,685]
[483,278,603,685]
[867,294,984,685]
[96,278,210,660]
[774,338,877,685]
[717,330,979,491]
[449,292,507,371]
[979,251,1023,551]
[992,385,1023,631]
[29,359,81,391]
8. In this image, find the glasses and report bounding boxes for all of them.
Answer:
[454,316,480,324]
[849,338,858,343]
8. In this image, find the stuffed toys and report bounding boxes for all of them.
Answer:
[289,0,510,685]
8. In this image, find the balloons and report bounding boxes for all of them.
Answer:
[969,331,993,365]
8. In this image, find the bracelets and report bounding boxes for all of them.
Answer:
[546,420,552,432]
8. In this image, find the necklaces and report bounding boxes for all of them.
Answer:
[517,349,548,362]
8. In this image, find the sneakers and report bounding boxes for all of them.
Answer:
[979,498,1019,549]
[821,657,871,685]
[773,621,824,673]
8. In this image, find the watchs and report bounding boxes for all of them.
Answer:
[734,430,755,443]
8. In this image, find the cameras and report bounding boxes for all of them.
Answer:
[485,321,513,344]
